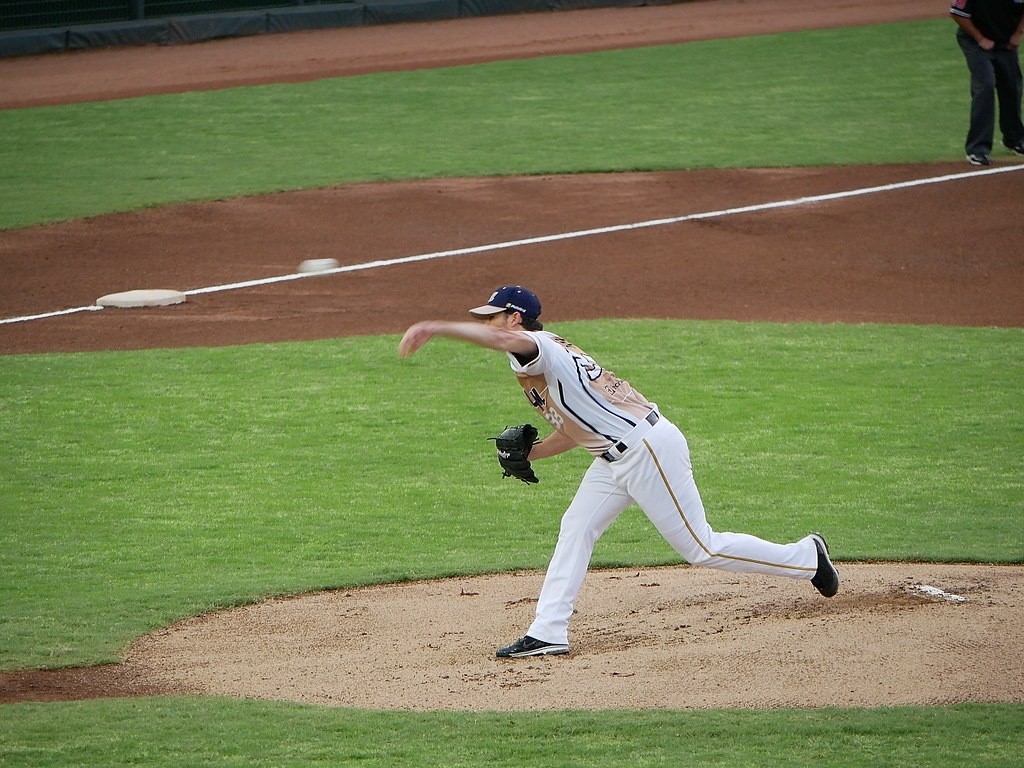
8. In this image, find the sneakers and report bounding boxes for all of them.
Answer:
[807,531,840,598]
[495,635,569,657]
[1000,136,1024,155]
[965,153,991,166]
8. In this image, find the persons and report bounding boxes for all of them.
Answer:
[947,0,1024,169]
[397,285,841,660]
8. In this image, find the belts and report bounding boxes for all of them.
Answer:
[600,412,660,462]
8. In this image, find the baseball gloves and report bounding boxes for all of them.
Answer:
[494,423,540,484]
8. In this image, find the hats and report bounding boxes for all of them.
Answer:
[468,286,542,320]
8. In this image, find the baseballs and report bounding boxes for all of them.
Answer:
[298,257,340,273]
